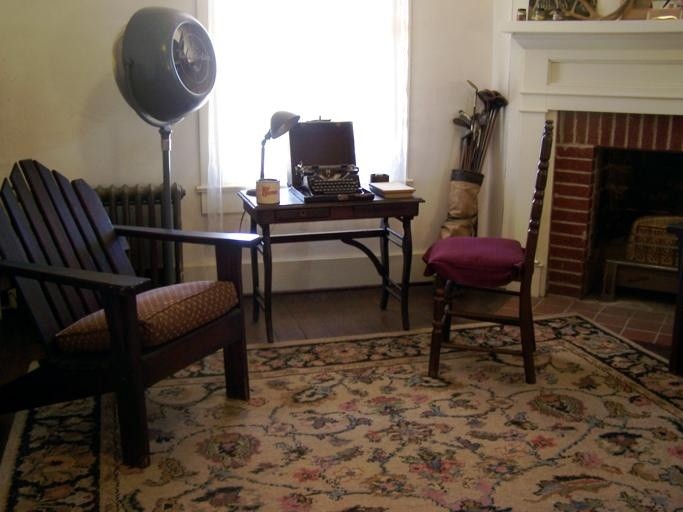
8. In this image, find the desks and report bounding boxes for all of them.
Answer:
[236,187,425,346]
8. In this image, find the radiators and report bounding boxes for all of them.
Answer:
[98,184,186,290]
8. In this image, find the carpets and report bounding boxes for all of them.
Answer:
[0,311,683,512]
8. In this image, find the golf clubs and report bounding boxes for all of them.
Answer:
[454,80,508,174]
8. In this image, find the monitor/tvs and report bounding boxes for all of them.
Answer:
[289,121,356,186]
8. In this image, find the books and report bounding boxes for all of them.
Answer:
[369,187,413,199]
[368,182,416,194]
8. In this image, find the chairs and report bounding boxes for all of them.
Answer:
[1,158,263,470]
[420,118,554,384]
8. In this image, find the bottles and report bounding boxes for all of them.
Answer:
[516,8,527,21]
[533,8,546,20]
[552,8,563,21]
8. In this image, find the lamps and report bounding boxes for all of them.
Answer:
[247,111,301,196]
